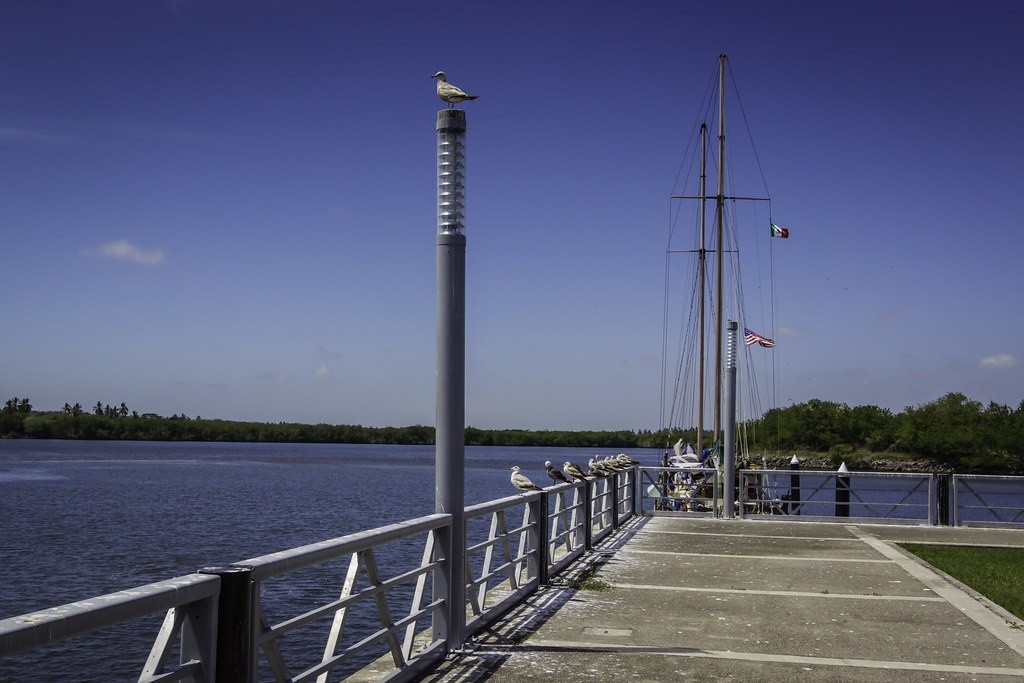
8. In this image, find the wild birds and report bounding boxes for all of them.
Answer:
[431,70,481,109]
[587,453,637,478]
[545,460,573,486]
[509,465,543,496]
[563,461,587,482]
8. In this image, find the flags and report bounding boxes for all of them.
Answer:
[744,328,775,348]
[770,223,789,239]
